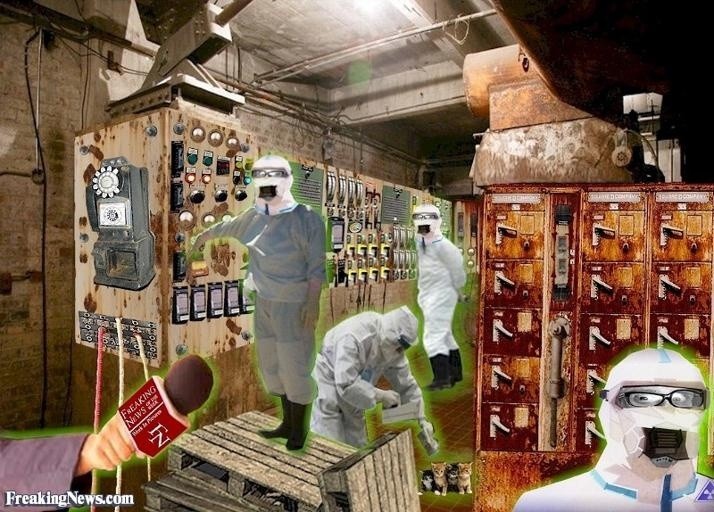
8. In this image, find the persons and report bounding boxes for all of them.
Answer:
[186,155,329,451]
[507,346,714,512]
[0,412,146,511]
[410,204,469,390]
[308,304,435,450]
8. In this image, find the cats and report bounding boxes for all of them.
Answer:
[417,460,473,497]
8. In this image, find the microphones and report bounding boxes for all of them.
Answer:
[117,355,214,460]
